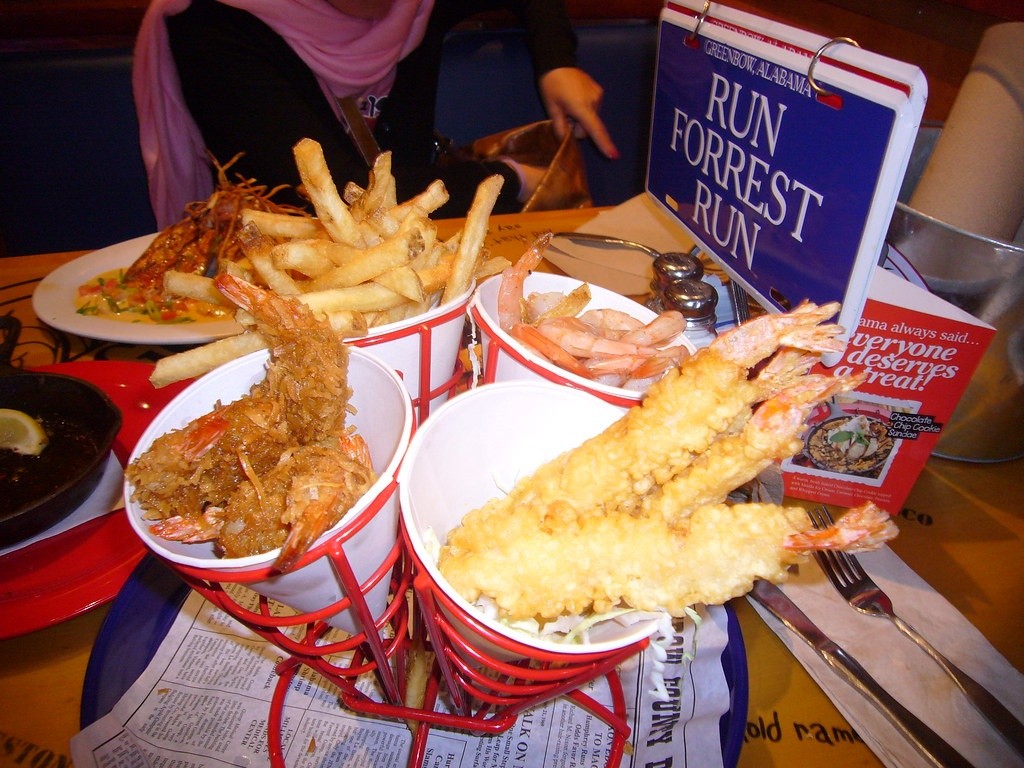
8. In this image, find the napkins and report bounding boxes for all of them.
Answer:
[744,540,1024,768]
[542,191,730,300]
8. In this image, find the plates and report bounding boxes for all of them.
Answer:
[31,231,245,347]
[0,359,198,640]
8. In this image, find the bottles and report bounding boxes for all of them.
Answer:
[646,252,704,315]
[658,279,718,351]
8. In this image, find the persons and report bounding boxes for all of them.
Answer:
[133,0,620,232]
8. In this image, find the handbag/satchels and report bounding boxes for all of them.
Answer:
[412,119,592,215]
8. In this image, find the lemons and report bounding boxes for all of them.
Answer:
[0,408,49,456]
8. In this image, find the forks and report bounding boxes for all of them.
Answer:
[807,504,1024,763]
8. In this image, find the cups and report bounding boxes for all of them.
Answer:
[339,278,477,431]
[123,346,414,641]
[398,380,675,713]
[473,271,698,401]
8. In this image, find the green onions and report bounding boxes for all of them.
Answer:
[77,269,194,325]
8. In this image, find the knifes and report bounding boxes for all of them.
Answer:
[747,579,976,768]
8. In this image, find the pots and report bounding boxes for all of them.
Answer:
[0,316,124,552]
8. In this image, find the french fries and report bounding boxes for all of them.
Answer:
[146,137,514,390]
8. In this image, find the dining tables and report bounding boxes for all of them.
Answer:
[0,209,1024,768]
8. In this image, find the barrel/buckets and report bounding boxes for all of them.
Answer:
[879,124,1024,464]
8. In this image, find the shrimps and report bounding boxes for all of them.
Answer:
[436,295,901,626]
[121,151,312,294]
[499,230,690,390]
[120,274,374,579]
